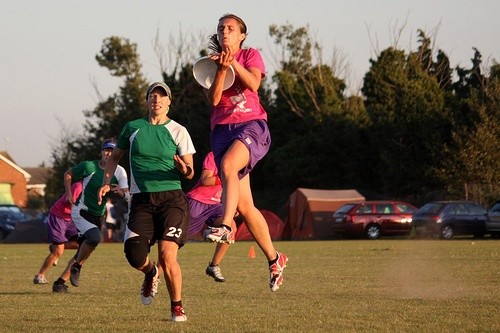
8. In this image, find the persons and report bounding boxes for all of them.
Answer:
[202,15,288,292]
[53,139,130,296]
[34,182,83,284]
[158,151,239,282]
[103,198,120,242]
[98,82,197,323]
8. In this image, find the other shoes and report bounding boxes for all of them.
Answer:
[206,262,225,282]
[52,281,69,293]
[70,263,80,287]
[33,274,50,284]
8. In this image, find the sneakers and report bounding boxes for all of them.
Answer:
[140,261,158,305]
[171,306,187,322]
[205,225,235,244]
[269,250,288,292]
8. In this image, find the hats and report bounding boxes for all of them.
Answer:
[146,81,172,101]
[102,137,118,150]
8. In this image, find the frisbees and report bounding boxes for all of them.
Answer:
[192,56,236,91]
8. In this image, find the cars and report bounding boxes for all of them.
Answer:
[413,199,488,240]
[487,202,500,239]
[0,204,28,239]
[331,200,419,240]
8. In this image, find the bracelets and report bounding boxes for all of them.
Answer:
[184,164,192,177]
